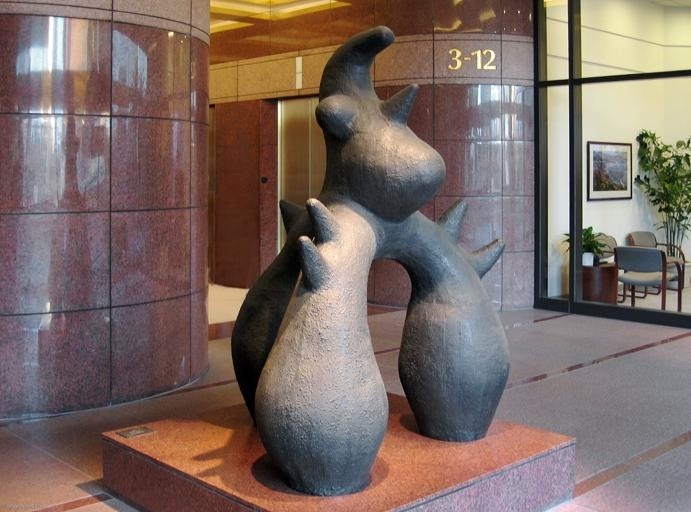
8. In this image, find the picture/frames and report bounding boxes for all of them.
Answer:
[587,140,632,201]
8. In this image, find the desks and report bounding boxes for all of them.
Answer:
[582,262,618,302]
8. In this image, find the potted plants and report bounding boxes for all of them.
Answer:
[565,225,603,266]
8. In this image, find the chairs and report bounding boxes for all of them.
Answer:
[614,246,683,313]
[628,231,685,295]
[590,230,647,303]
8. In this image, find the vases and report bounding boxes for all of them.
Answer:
[668,260,691,290]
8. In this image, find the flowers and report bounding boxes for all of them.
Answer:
[633,128,691,262]
